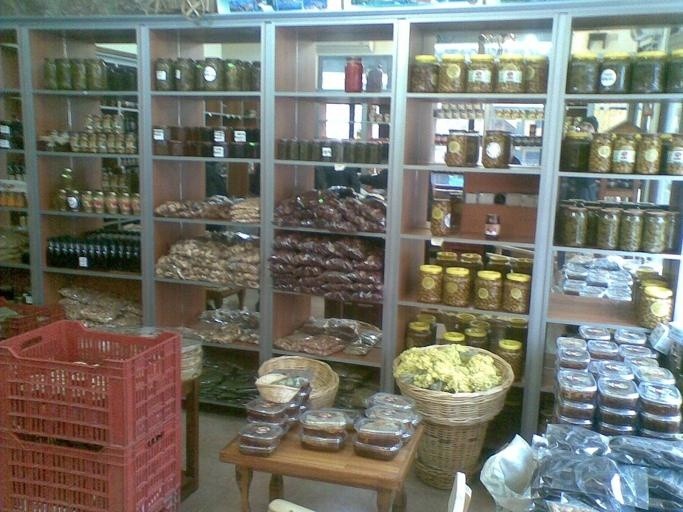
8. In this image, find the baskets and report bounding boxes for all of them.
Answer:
[258,356,341,410]
[0,297,182,511]
[392,344,515,491]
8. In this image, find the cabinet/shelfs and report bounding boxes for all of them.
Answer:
[181,376,199,498]
[0,14,683,453]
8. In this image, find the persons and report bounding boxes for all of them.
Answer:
[358,168,434,262]
[562,116,597,200]
[314,167,361,194]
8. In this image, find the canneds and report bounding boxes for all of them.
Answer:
[468,55,495,92]
[439,55,468,91]
[403,250,533,383]
[153,111,260,157]
[157,57,261,91]
[566,51,683,93]
[554,197,679,252]
[485,213,501,239]
[42,57,133,90]
[632,269,674,327]
[410,55,440,93]
[0,119,23,150]
[560,130,683,176]
[430,198,452,236]
[526,57,549,92]
[495,54,526,92]
[344,57,364,92]
[445,130,510,168]
[52,163,140,217]
[275,138,389,164]
[36,115,138,153]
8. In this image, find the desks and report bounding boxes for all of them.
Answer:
[218,421,424,512]
